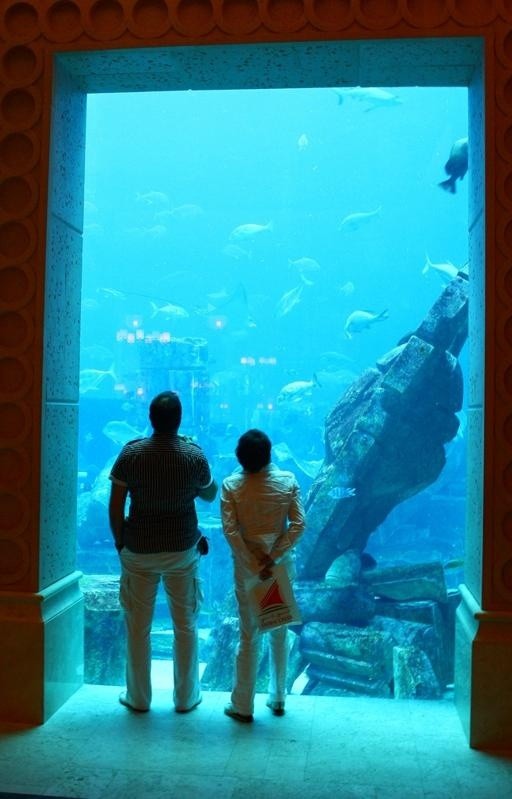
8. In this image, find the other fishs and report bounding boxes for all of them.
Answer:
[79,189,317,482]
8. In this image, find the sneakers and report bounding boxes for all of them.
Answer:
[119,691,150,712]
[223,706,252,721]
[175,695,203,714]
[266,701,284,716]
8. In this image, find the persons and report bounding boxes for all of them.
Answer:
[108,389,220,714]
[220,428,309,723]
